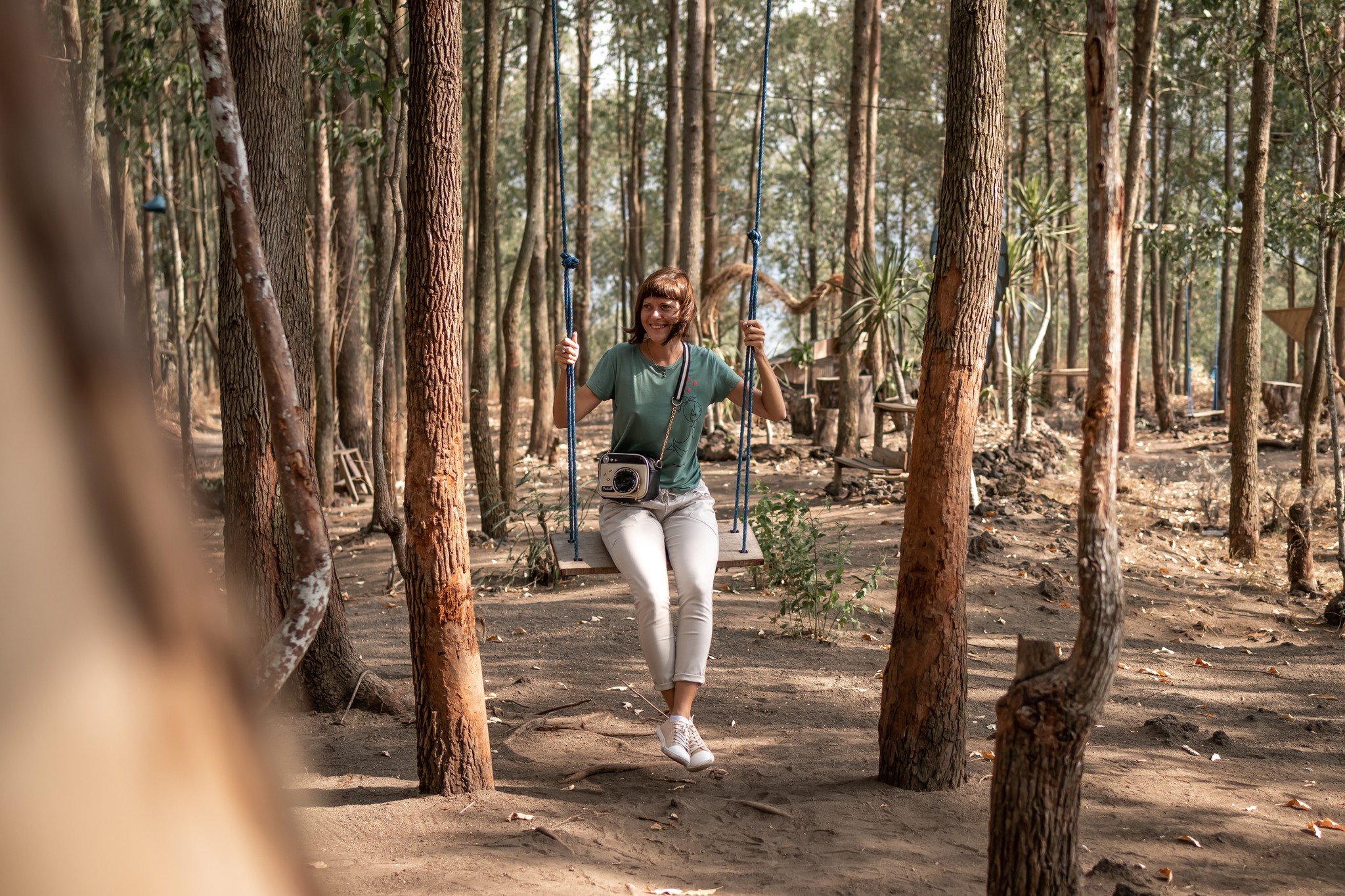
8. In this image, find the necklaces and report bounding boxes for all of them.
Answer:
[647,339,683,377]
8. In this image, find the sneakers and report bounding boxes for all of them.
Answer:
[683,717,715,772]
[655,714,698,768]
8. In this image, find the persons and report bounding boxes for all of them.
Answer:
[550,268,787,771]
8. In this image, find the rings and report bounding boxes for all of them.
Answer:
[561,345,568,354]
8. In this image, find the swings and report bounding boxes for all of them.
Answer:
[545,2,770,575]
[1177,104,1227,421]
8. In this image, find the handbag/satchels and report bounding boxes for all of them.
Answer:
[597,452,662,503]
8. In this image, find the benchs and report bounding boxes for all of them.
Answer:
[833,400,916,495]
